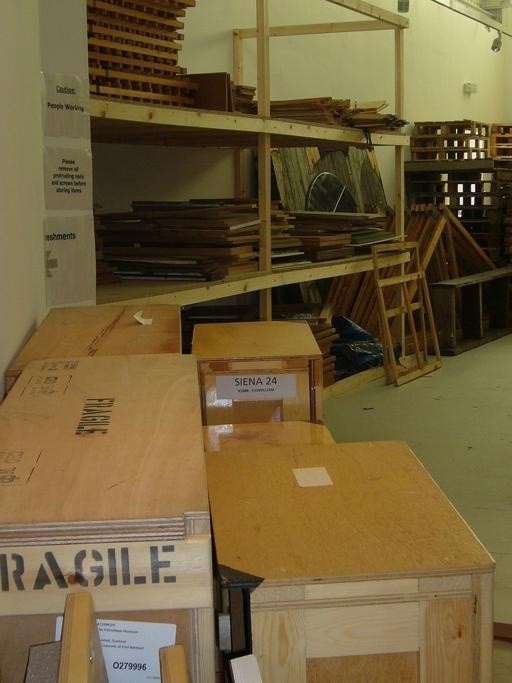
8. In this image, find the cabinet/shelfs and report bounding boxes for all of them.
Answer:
[84,0,408,389]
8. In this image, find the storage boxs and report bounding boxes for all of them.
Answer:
[205,439,496,682]
[189,319,326,422]
[5,305,182,391]
[2,353,217,683]
